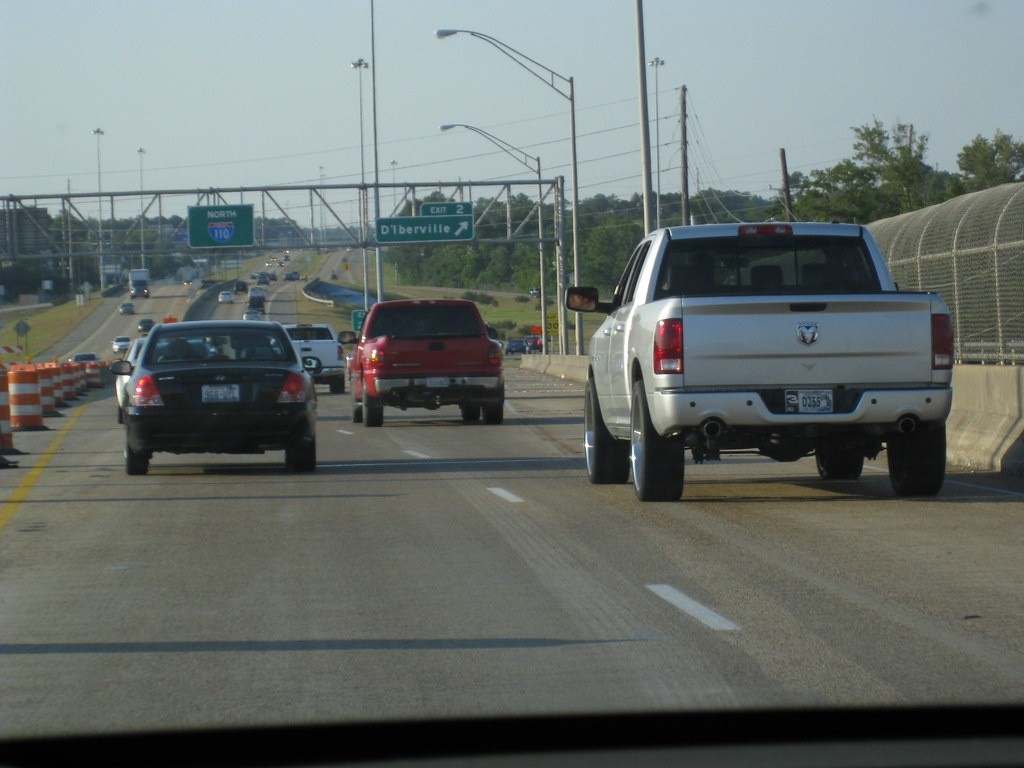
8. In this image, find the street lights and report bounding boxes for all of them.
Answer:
[349,58,369,311]
[433,28,585,356]
[135,148,147,270]
[92,127,108,289]
[439,123,549,355]
[648,56,664,230]
[390,159,398,217]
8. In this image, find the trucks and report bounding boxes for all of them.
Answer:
[128,268,151,300]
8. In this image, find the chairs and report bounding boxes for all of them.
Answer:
[668,265,708,291]
[749,264,784,290]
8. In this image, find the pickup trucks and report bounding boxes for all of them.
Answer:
[561,219,956,503]
[337,299,506,428]
[270,323,348,394]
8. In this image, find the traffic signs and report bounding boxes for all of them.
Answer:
[373,201,476,244]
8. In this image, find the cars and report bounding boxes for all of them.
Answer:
[137,319,155,334]
[504,335,542,355]
[118,302,135,315]
[110,336,131,354]
[218,254,301,322]
[110,337,220,424]
[68,352,97,363]
[109,318,322,475]
